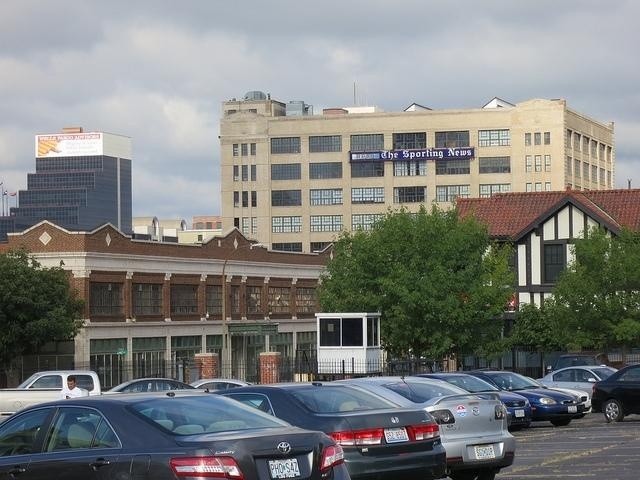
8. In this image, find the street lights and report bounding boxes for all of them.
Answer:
[220,242,272,379]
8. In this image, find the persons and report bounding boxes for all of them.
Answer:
[58,375,83,398]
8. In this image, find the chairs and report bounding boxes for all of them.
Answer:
[68,422,100,448]
[335,395,361,412]
[153,417,247,435]
